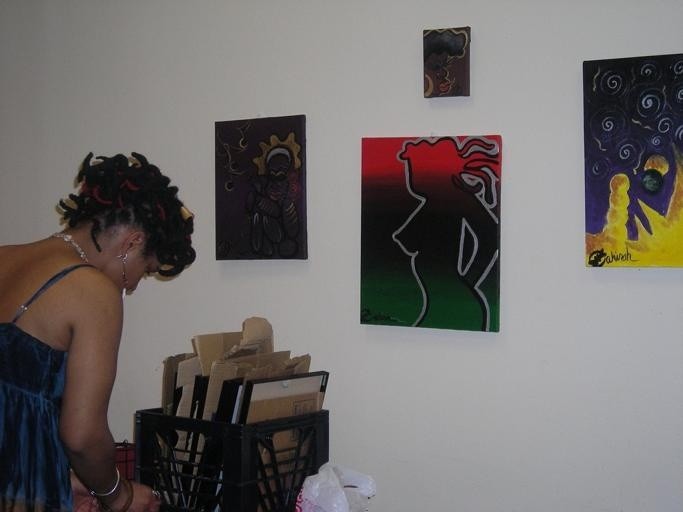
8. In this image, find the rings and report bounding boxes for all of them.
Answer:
[152,490,160,498]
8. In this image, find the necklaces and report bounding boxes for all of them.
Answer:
[53,233,89,263]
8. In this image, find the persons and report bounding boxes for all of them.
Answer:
[0,152,195,512]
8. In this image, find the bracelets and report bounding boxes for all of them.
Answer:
[94,479,133,512]
[89,467,120,497]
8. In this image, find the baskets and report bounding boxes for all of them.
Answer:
[134,408,329,511]
